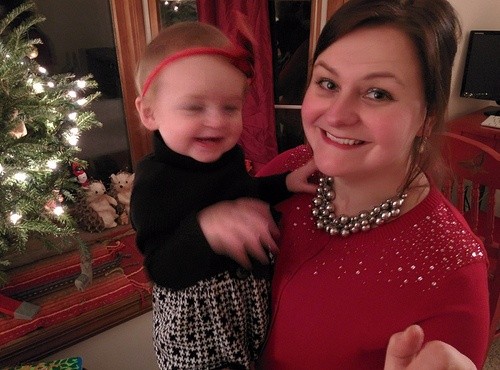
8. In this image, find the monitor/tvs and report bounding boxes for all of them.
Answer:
[460,30,500,116]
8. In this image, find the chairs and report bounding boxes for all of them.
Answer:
[418,131,500,370]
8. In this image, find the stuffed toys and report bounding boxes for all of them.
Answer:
[110,171,136,214]
[71,162,88,191]
[85,179,119,228]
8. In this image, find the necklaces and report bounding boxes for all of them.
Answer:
[309,174,408,235]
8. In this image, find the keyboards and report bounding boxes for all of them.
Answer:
[481,115,500,129]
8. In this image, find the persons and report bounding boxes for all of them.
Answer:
[253,0,492,370]
[128,19,321,370]
